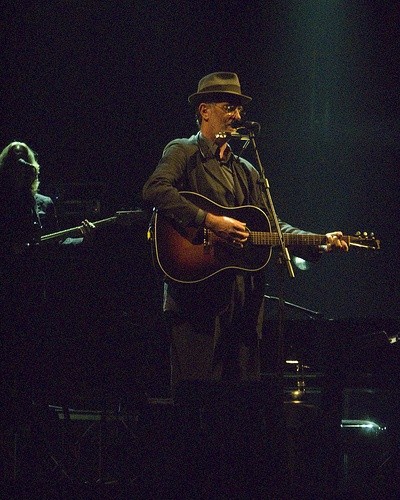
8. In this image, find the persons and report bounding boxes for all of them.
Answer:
[143,73,349,401]
[0,141,96,481]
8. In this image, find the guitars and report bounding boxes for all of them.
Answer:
[0,205,152,278]
[149,189,381,286]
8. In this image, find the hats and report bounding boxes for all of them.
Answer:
[188,72,252,106]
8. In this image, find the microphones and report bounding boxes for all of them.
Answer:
[231,119,260,133]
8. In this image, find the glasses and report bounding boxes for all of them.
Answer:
[209,100,243,113]
